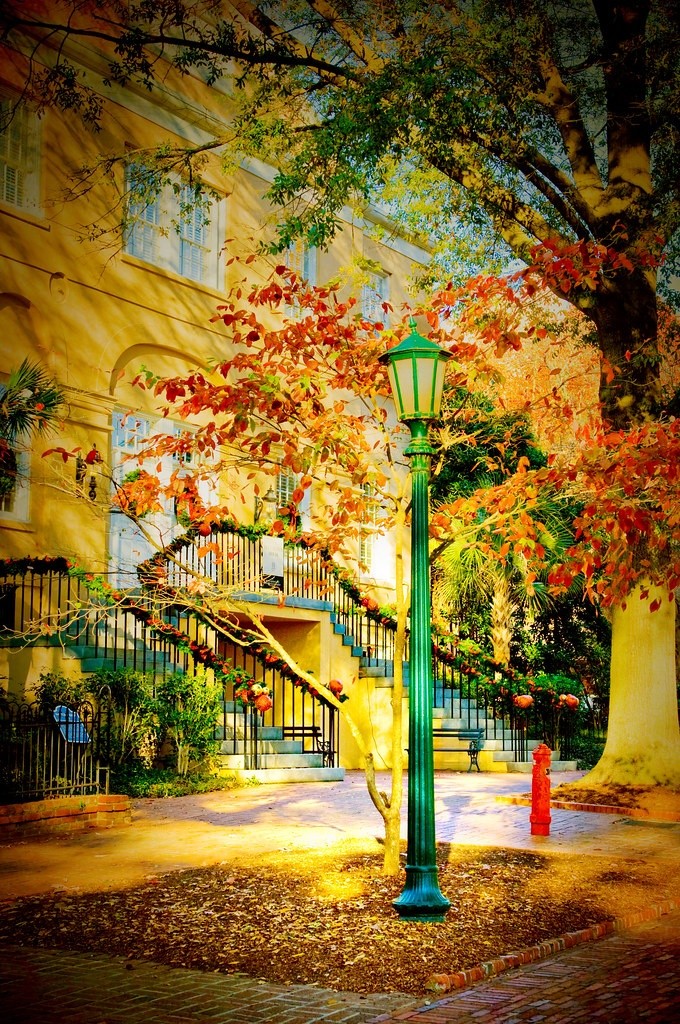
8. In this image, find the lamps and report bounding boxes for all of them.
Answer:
[76,442,105,502]
[254,484,278,526]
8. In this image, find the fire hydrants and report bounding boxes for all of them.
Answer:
[527,742,554,836]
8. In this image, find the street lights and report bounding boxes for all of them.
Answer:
[374,316,457,927]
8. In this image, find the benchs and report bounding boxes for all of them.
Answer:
[275,727,336,768]
[405,728,484,773]
